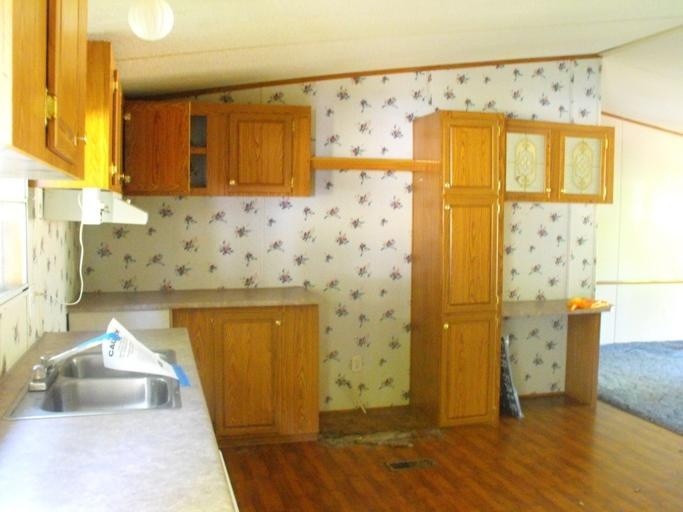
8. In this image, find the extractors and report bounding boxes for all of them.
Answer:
[42,187,148,225]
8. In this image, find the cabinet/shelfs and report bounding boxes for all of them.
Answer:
[0,2,122,191]
[124,101,312,199]
[501,118,614,202]
[415,113,502,430]
[174,303,317,449]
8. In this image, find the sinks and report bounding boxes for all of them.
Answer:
[56,343,176,379]
[5,378,181,413]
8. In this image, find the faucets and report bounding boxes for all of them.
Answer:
[41,332,120,361]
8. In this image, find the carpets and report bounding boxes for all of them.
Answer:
[598,342,683,440]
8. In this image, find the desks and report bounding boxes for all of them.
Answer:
[504,299,613,408]
